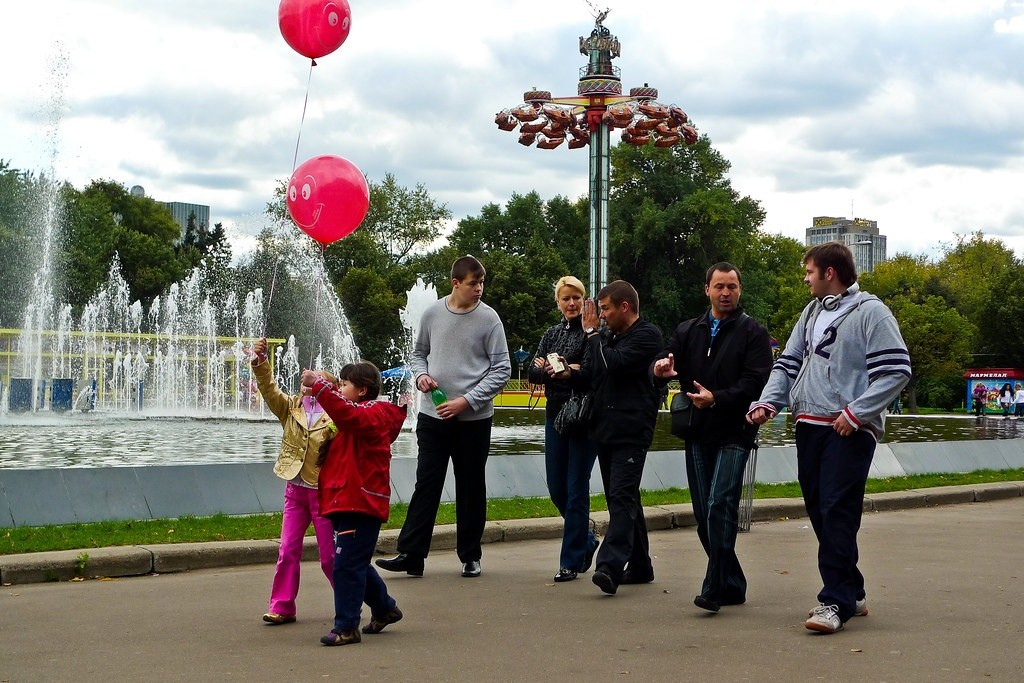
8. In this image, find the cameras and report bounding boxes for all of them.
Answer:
[547,352,565,373]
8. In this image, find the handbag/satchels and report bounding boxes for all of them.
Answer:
[971,394,980,399]
[670,391,700,438]
[553,390,599,449]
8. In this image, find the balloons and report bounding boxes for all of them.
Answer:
[278,0,351,66]
[286,154,370,251]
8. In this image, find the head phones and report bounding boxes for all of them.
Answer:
[816,281,859,311]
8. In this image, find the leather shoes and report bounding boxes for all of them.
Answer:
[461,559,481,577]
[554,568,577,582]
[578,540,599,573]
[375,554,424,576]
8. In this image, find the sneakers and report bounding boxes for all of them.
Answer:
[809,595,868,616]
[362,606,403,634]
[320,629,361,645]
[805,604,844,632]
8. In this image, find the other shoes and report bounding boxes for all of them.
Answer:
[693,595,720,612]
[263,612,296,624]
[717,595,745,606]
[591,571,617,595]
[621,563,654,585]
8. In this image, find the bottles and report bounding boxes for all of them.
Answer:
[431,386,447,406]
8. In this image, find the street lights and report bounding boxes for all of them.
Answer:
[846,240,872,248]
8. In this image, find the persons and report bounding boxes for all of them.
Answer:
[648,261,774,612]
[527,276,664,593]
[658,384,669,410]
[376,255,512,576]
[745,241,912,631]
[250,336,335,622]
[971,382,988,415]
[302,361,408,645]
[1010,382,1024,416]
[890,393,902,415]
[997,382,1015,416]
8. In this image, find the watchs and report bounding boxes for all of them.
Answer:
[583,326,598,338]
[709,401,717,409]
